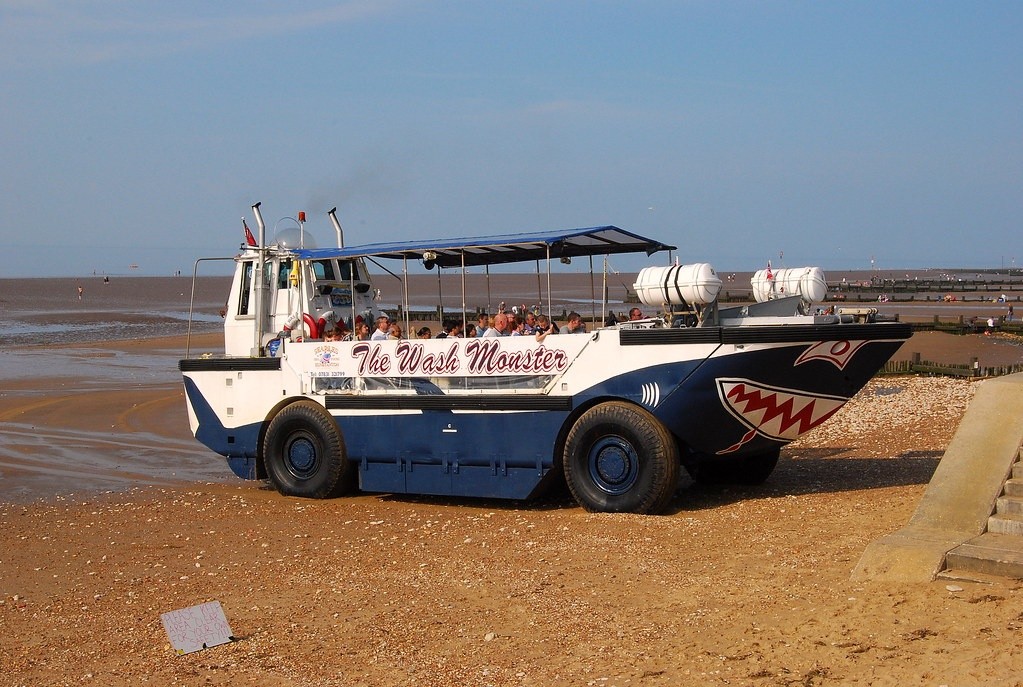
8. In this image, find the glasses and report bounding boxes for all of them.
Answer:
[637,313,642,316]
[380,320,389,324]
[424,332,432,335]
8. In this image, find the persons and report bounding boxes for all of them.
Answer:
[371,317,390,339]
[938,293,956,302]
[559,312,584,334]
[78,286,83,299]
[627,308,643,321]
[436,311,560,339]
[388,325,402,340]
[417,327,432,339]
[353,321,369,340]
[877,291,888,303]
[821,306,834,315]
[104,275,109,285]
[967,316,978,327]
[581,322,587,332]
[606,310,616,326]
[388,318,397,329]
[992,293,1007,304]
[986,316,995,335]
[1007,303,1014,321]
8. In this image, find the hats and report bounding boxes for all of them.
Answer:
[501,302,505,305]
[447,320,459,330]
